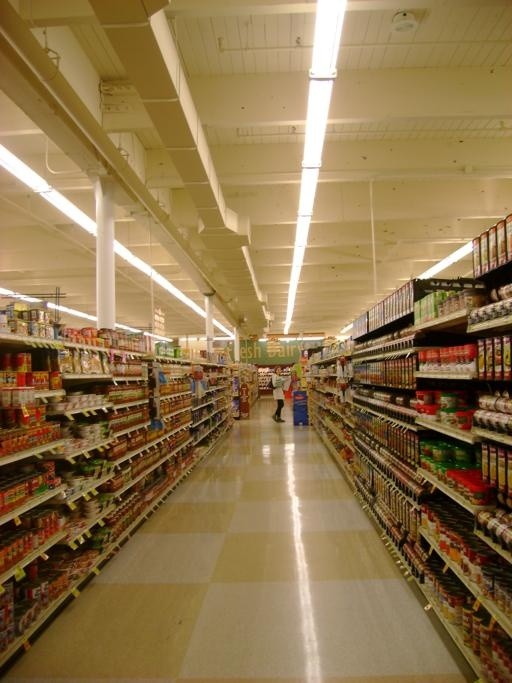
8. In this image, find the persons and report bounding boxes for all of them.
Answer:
[272,366,285,422]
